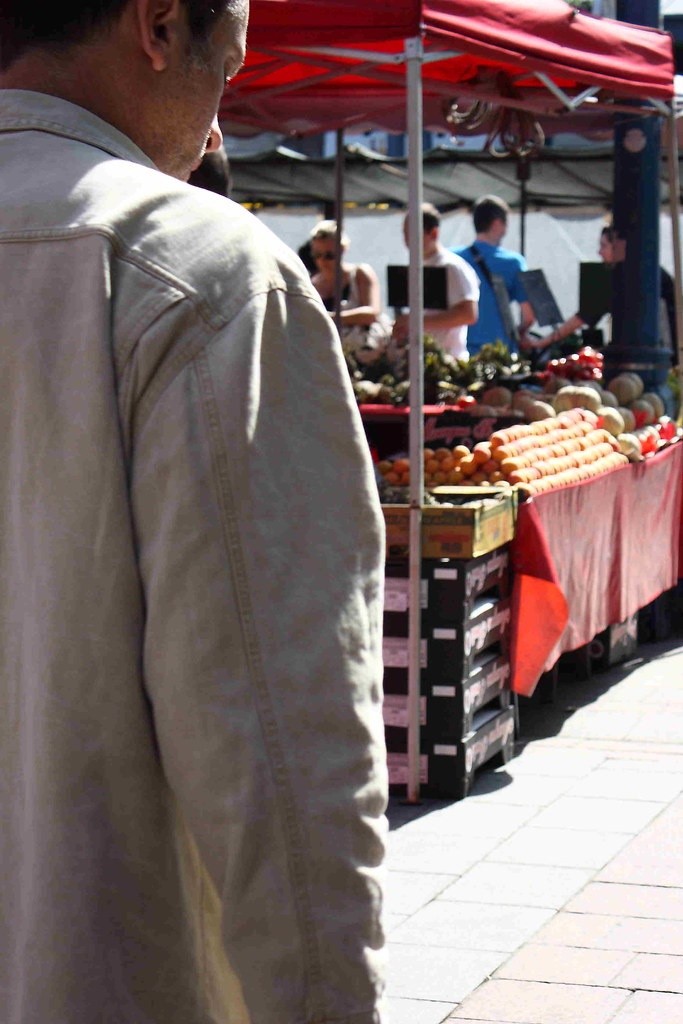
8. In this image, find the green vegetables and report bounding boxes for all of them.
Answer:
[339,331,515,405]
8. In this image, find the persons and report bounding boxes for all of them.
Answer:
[391,201,483,362]
[447,194,535,360]
[517,226,680,420]
[308,220,380,327]
[186,145,234,202]
[1,0,389,1024]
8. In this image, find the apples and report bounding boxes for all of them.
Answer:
[375,410,630,500]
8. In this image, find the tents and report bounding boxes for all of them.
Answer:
[220,0,682,802]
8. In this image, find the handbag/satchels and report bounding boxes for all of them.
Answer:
[491,277,520,342]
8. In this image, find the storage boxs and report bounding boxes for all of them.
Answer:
[610,613,636,668]
[383,486,518,802]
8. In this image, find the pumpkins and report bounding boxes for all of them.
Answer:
[483,371,676,457]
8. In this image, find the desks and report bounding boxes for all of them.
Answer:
[510,441,683,698]
[359,401,535,462]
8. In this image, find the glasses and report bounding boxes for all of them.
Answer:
[312,250,336,261]
